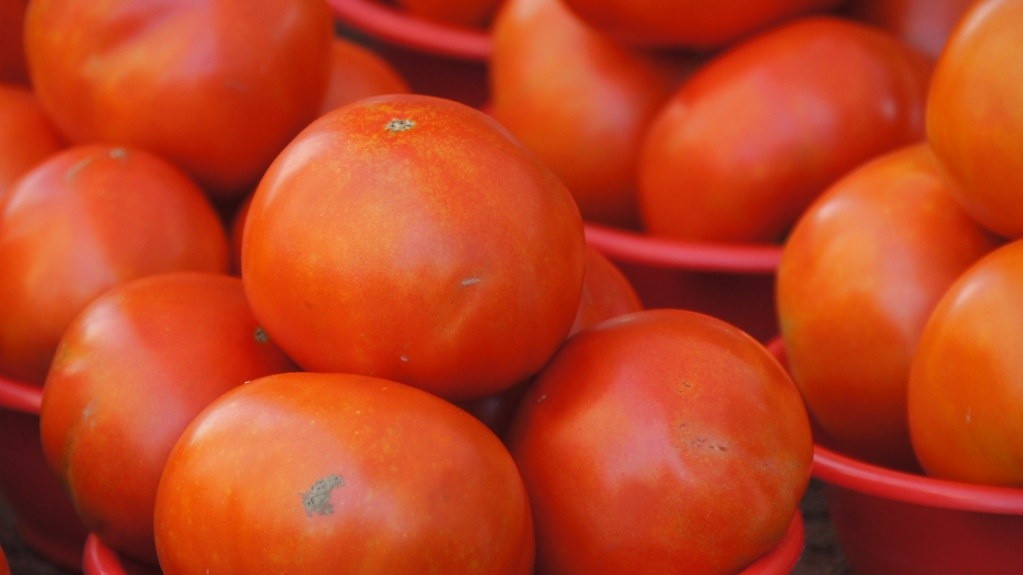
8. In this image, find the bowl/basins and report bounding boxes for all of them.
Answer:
[760,332,1022,573]
[79,506,811,574]
[574,223,800,344]
[0,376,90,567]
[332,1,502,110]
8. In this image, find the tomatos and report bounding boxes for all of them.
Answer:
[0,0,1023,574]
